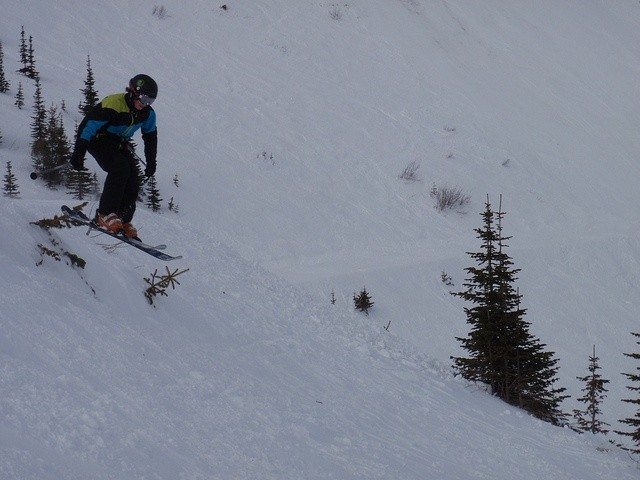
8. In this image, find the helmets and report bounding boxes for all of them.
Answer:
[129,74,158,106]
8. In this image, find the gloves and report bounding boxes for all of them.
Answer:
[70,152,88,171]
[145,154,156,177]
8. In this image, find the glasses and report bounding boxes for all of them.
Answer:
[139,95,156,105]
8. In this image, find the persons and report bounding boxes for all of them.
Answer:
[70,74,158,237]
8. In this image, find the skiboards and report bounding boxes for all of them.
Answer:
[62,206,183,261]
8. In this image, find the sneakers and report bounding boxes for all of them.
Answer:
[93,209,126,235]
[122,221,137,238]
